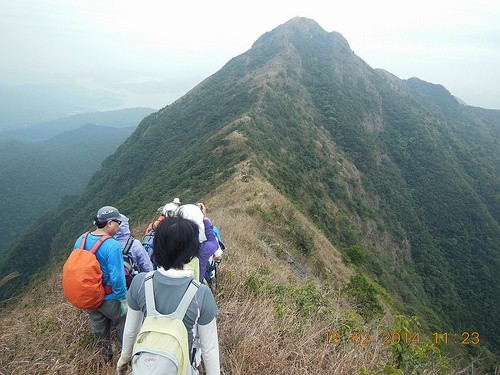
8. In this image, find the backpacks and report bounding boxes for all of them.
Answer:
[62,233,112,310]
[131,273,201,375]
[121,238,141,290]
[141,223,157,265]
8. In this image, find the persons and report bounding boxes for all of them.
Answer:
[74,206,133,375]
[113,213,153,291]
[117,207,220,375]
[207,237,223,292]
[173,198,180,206]
[150,202,219,283]
[145,202,179,235]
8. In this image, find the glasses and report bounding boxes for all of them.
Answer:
[112,220,122,225]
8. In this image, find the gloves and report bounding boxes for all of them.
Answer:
[117,357,130,375]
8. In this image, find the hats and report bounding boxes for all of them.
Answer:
[97,206,130,223]
[173,198,180,203]
